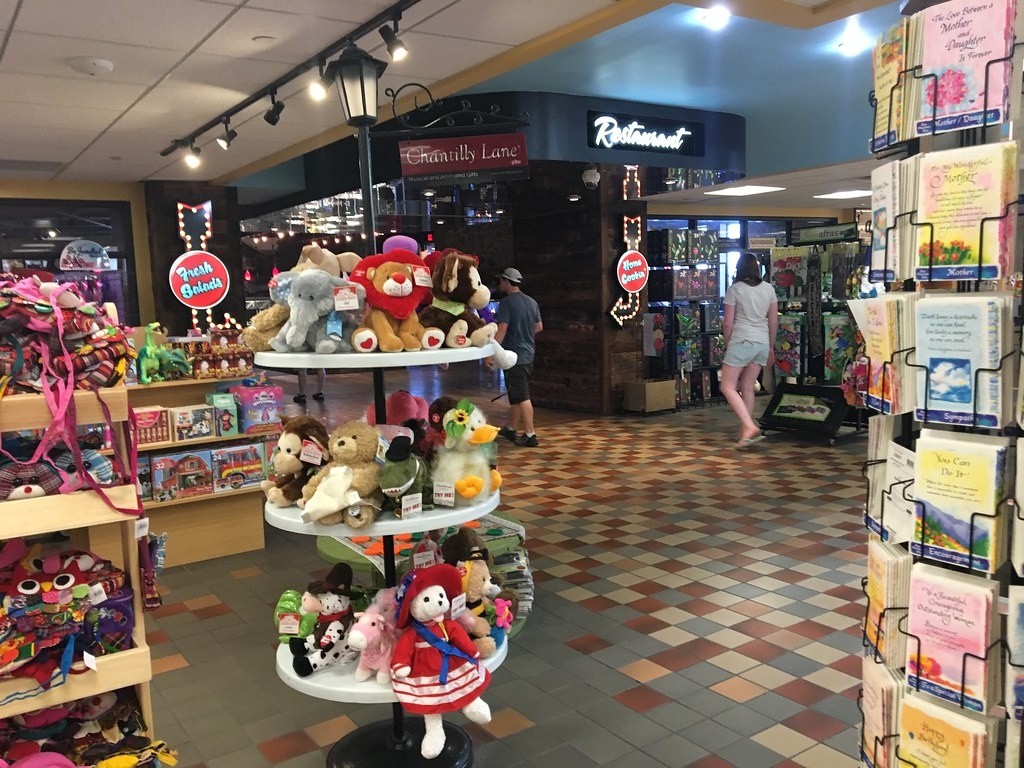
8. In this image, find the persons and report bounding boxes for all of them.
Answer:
[494,265,544,444]
[719,254,778,449]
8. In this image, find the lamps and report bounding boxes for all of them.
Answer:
[185,138,201,168]
[309,57,334,101]
[216,115,238,152]
[379,12,409,62]
[263,87,285,127]
[325,38,475,768]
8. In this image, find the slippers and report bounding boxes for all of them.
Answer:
[734,435,766,451]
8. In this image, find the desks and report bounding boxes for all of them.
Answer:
[315,508,524,589]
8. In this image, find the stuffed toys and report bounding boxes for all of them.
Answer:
[241,233,518,761]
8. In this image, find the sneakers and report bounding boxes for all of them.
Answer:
[497,424,516,442]
[513,432,539,447]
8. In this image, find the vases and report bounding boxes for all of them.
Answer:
[717,371,722,381]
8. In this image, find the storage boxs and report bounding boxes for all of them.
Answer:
[622,378,677,414]
[213,442,264,492]
[124,337,139,388]
[164,327,253,381]
[127,404,172,448]
[204,392,239,437]
[267,440,285,480]
[150,448,213,502]
[137,452,152,502]
[650,227,727,402]
[172,403,216,442]
[230,384,284,432]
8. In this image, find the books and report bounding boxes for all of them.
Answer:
[845,0,1024,768]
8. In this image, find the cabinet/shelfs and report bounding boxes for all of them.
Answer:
[253,343,511,768]
[756,221,869,445]
[70,328,266,569]
[0,379,157,744]
[647,260,725,411]
[856,0,1024,768]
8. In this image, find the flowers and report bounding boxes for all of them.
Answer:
[713,334,727,370]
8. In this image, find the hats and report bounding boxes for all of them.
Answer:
[494,268,522,283]
